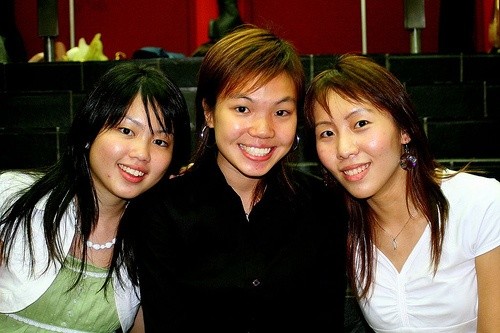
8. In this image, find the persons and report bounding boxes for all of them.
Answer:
[305,53,500,333]
[0,63,196,333]
[127,24,352,333]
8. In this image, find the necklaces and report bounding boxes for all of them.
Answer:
[366,206,419,250]
[74,211,117,250]
